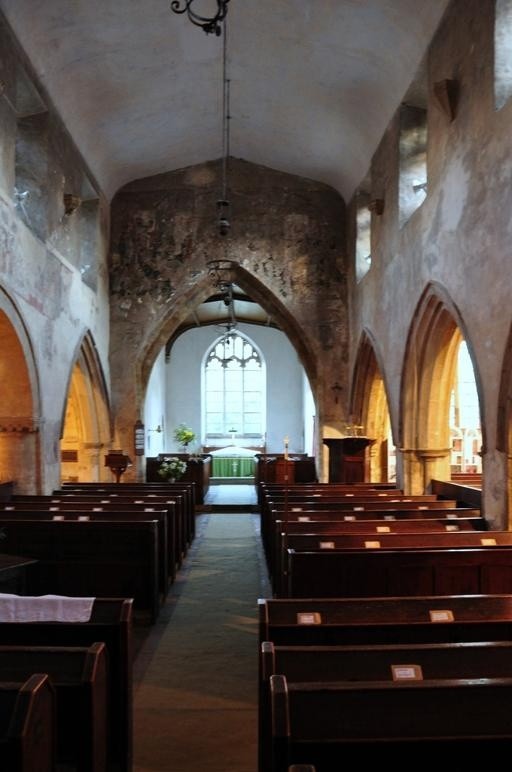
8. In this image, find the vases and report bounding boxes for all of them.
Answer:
[183,446,188,453]
[168,476,176,483]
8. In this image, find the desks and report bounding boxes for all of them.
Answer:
[209,454,256,477]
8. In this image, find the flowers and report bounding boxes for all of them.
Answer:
[174,422,195,445]
[157,456,187,479]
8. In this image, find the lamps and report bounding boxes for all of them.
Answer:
[205,14,240,329]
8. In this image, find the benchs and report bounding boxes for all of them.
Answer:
[257,482,512,772]
[0,481,195,772]
